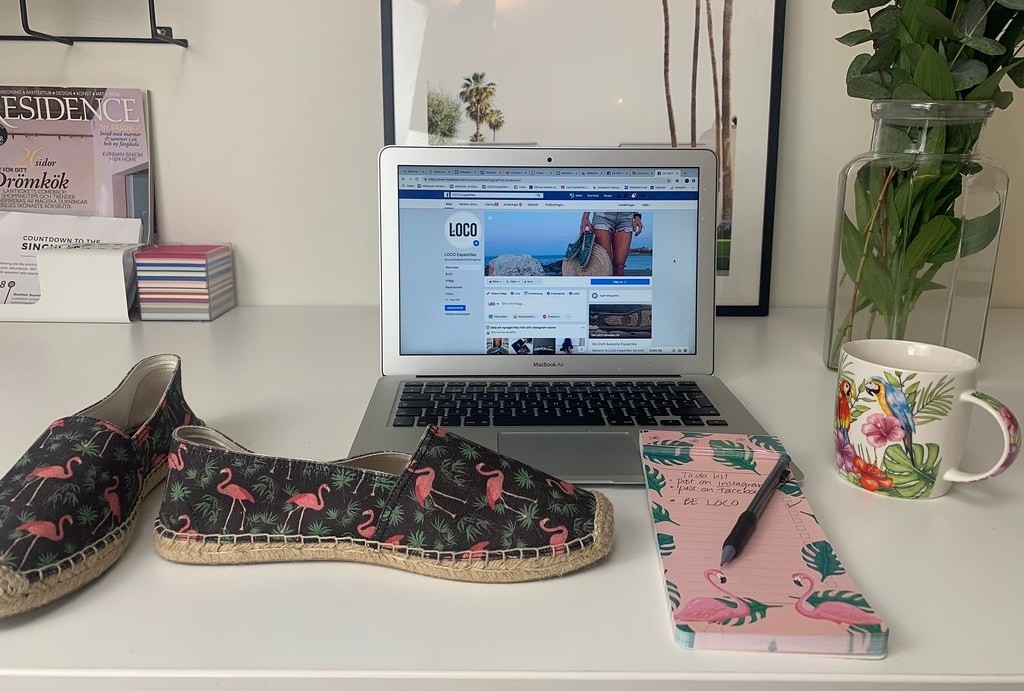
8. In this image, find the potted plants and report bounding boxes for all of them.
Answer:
[821,0,1023,375]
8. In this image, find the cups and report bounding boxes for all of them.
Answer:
[830,336,1024,503]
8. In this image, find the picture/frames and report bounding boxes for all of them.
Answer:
[379,0,788,319]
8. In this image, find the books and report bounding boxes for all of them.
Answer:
[636,430,890,663]
[1,84,156,326]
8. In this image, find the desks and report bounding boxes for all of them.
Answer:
[3,307,1024,689]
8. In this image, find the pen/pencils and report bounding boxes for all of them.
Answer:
[720,453,791,567]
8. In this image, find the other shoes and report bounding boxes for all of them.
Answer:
[2,354,203,624]
[596,308,651,332]
[150,422,617,584]
[580,233,595,268]
[564,231,589,260]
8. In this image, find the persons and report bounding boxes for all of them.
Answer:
[580,212,643,276]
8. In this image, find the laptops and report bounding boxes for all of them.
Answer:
[348,144,805,486]
